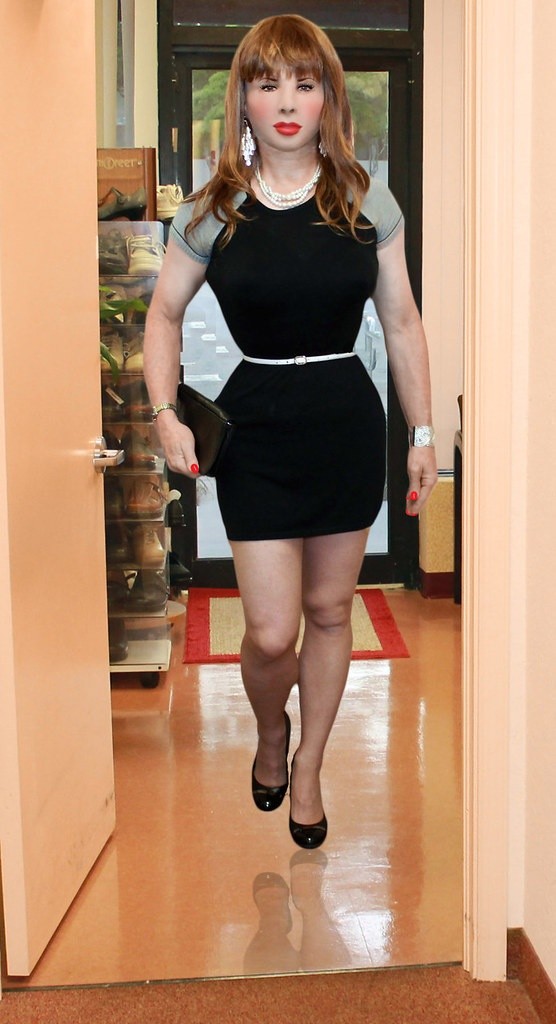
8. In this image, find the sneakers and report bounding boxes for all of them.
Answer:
[98,226,167,276]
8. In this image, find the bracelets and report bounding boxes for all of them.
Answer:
[407,426,436,449]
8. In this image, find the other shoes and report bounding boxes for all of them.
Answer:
[100,327,144,373]
[104,476,167,519]
[103,425,158,470]
[166,499,189,528]
[107,571,170,612]
[157,182,185,225]
[102,379,151,423]
[106,524,165,566]
[109,618,130,661]
[168,550,193,587]
[99,282,152,325]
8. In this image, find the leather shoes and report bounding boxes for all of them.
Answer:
[290,848,328,871]
[289,813,328,849]
[252,873,293,936]
[252,711,291,812]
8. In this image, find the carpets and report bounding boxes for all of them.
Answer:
[0,962,539,1024]
[182,586,411,665]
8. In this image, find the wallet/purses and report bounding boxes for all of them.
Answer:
[177,383,235,476]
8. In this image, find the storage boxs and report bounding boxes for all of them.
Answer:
[98,219,171,663]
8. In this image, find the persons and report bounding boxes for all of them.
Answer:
[139,12,438,851]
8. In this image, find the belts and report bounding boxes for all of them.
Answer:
[243,350,356,366]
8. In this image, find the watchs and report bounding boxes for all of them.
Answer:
[150,402,179,423]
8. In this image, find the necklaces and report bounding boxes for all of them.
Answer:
[253,157,323,209]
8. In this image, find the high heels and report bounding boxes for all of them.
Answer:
[98,185,147,221]
